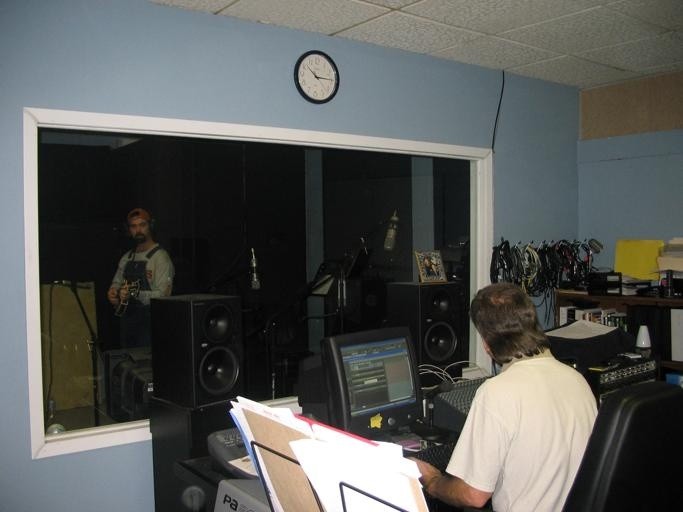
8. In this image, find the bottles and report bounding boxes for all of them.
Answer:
[634,325,653,348]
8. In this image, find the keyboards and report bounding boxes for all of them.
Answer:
[408,441,456,475]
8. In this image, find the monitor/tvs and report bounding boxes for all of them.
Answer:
[321,326,425,435]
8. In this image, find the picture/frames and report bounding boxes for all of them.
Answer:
[414,249,448,283]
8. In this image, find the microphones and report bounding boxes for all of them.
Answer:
[61,280,91,291]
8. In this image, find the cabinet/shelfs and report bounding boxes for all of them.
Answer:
[553,280,683,389]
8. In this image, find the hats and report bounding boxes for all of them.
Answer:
[127,208,151,223]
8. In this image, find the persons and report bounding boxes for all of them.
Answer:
[408,283,600,511]
[98,208,175,355]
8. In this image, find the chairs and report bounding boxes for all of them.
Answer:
[552,377,682,511]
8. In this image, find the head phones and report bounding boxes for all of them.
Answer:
[123,206,157,234]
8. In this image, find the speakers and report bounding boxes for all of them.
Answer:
[149,293,245,414]
[387,281,457,390]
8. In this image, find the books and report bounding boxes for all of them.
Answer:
[557,303,631,335]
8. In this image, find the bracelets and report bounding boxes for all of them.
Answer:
[421,475,446,493]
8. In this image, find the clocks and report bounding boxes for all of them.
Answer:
[294,49,339,104]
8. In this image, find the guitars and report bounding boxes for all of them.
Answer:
[111,278,141,315]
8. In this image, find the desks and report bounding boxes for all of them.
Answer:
[173,413,460,511]
